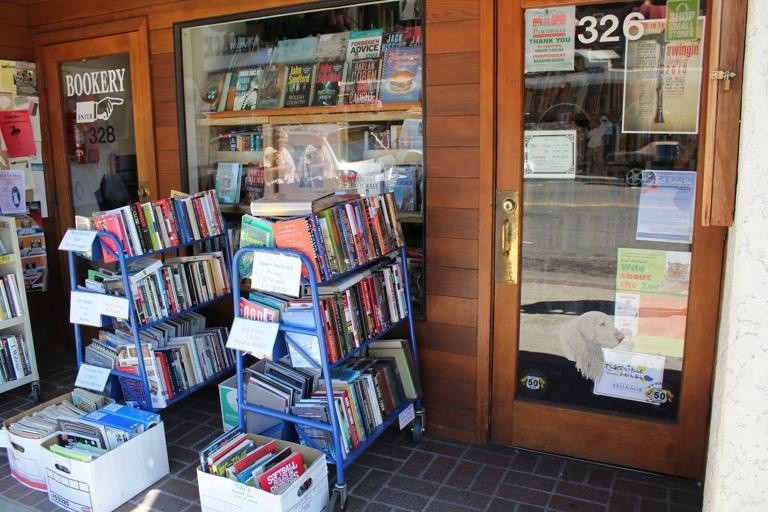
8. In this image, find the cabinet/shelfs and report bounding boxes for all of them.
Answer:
[196,107,425,425]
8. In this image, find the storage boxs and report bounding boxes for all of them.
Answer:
[1,390,116,493]
[195,433,331,512]
[38,402,171,511]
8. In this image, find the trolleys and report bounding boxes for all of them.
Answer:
[0,215,42,406]
[65,222,246,412]
[229,239,429,512]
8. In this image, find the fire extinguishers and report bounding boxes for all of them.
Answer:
[68,110,87,164]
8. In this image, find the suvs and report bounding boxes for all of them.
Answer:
[197,121,403,205]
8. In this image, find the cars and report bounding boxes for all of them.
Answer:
[603,139,697,189]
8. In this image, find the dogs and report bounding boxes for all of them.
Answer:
[559,310,625,385]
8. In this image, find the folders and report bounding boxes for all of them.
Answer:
[80,402,161,440]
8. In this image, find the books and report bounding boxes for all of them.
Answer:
[198,426,305,495]
[1,242,32,383]
[75,189,236,400]
[0,168,28,214]
[6,388,161,463]
[197,25,425,183]
[238,188,422,459]
[522,54,698,170]
